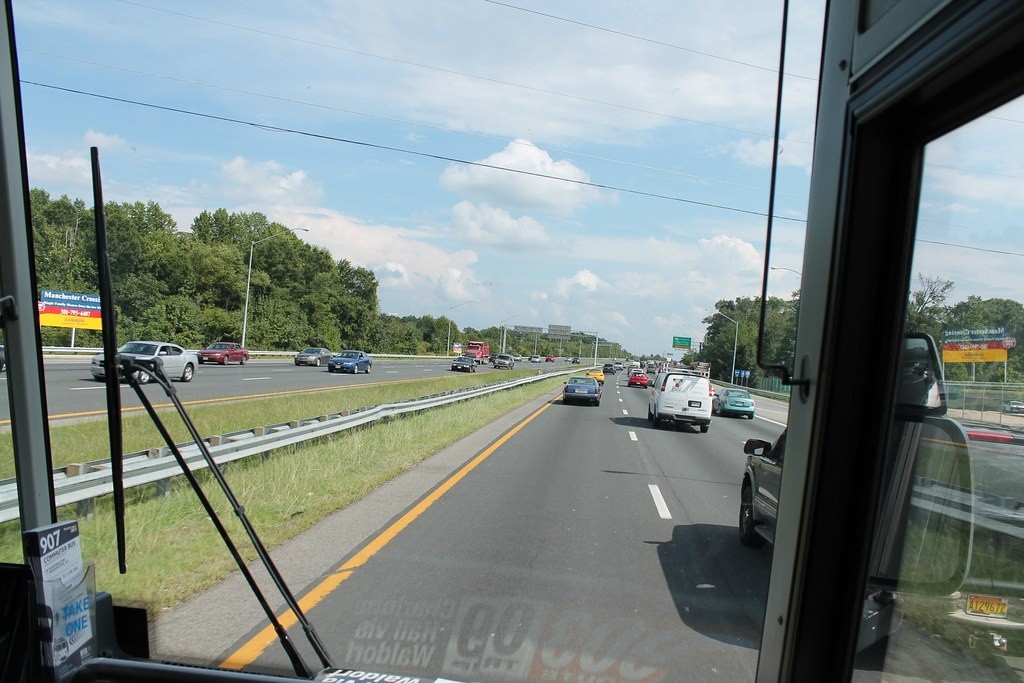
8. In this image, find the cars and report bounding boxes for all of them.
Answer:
[584,358,711,388]
[563,376,602,408]
[294,348,334,367]
[197,341,250,366]
[0,347,6,373]
[328,350,373,374]
[565,357,572,362]
[489,354,540,369]
[91,340,198,385]
[713,388,755,419]
[546,355,555,362]
[450,357,477,372]
[1001,400,1024,413]
[572,357,580,364]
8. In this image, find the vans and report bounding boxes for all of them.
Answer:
[647,371,714,434]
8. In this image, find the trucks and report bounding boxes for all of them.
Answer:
[465,341,490,365]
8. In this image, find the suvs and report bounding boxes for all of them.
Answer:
[737,413,1023,657]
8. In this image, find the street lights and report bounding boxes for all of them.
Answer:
[447,300,479,356]
[242,228,309,349]
[683,306,739,384]
[499,314,607,358]
[570,330,598,367]
